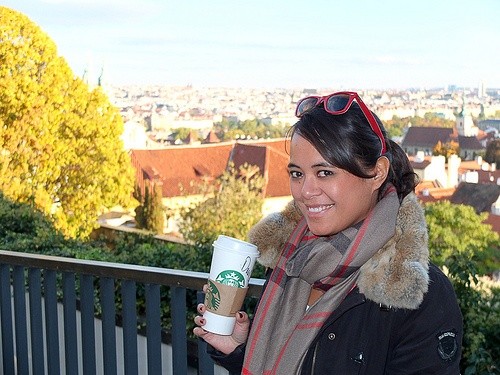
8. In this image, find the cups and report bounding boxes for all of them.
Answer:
[200,235,261,335]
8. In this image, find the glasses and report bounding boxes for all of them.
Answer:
[294,93,387,156]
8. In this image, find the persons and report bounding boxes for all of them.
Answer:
[193,97,464,374]
